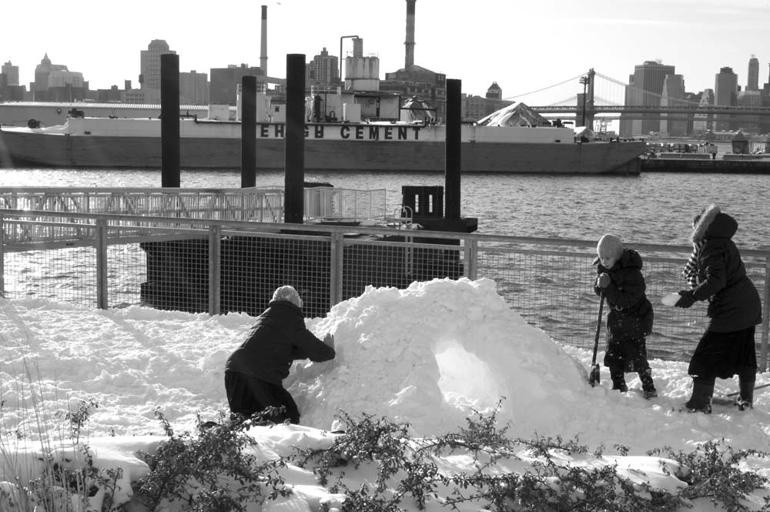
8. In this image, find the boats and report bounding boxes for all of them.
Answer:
[0,34,648,173]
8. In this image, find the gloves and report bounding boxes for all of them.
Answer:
[675,290,695,308]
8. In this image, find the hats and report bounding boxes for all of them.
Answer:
[273,285,302,308]
[596,234,623,258]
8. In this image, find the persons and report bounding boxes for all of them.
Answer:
[591,231,660,399]
[222,285,337,424]
[670,202,764,416]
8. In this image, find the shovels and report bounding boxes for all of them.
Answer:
[712,383,770,405]
[588,290,605,388]
